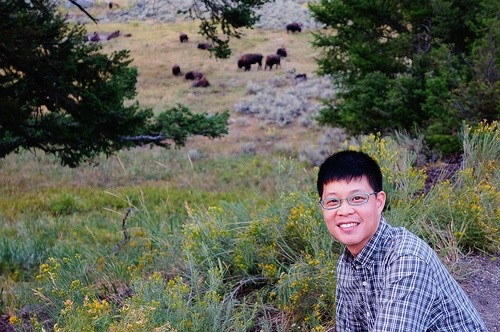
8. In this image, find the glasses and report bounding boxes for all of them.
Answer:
[319,192,377,210]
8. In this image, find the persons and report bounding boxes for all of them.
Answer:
[317,150,490,332]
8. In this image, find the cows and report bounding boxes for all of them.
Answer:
[184,70,212,88]
[106,30,120,40]
[89,31,101,43]
[264,54,281,71]
[178,31,188,43]
[172,64,181,76]
[276,47,287,58]
[237,54,264,71]
[286,22,303,34]
[197,42,213,50]
[123,32,132,37]
